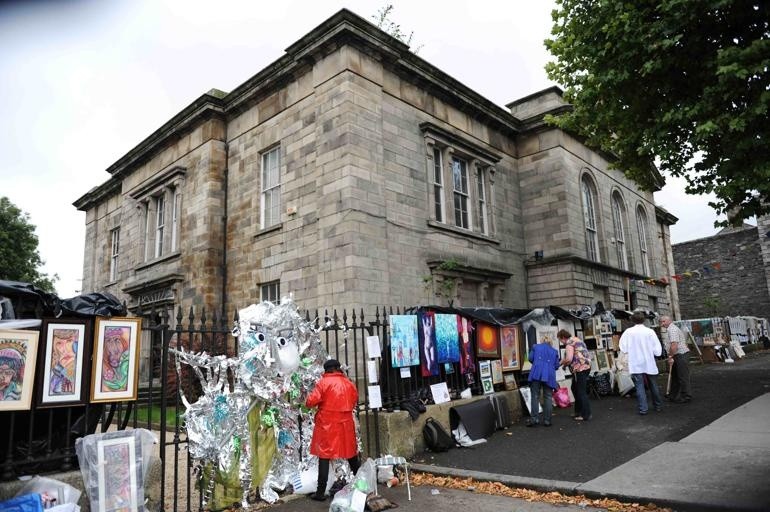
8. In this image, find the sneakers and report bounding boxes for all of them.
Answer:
[571,413,592,421]
[544,419,551,426]
[311,493,326,501]
[525,417,539,426]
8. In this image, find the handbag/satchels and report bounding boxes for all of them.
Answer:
[593,371,611,395]
[366,492,396,511]
[615,370,635,397]
[554,386,570,408]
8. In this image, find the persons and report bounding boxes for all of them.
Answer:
[658,315,692,404]
[523,335,560,427]
[457,315,475,371]
[102,327,129,392]
[407,347,415,366]
[305,359,359,502]
[618,310,663,415]
[421,314,435,373]
[0,347,22,401]
[396,340,406,367]
[50,328,78,394]
[556,328,593,422]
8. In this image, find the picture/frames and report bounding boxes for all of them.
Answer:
[474,315,625,396]
[36,318,91,410]
[90,315,142,404]
[90,430,145,512]
[0,328,41,411]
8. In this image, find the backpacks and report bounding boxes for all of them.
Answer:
[423,416,457,452]
